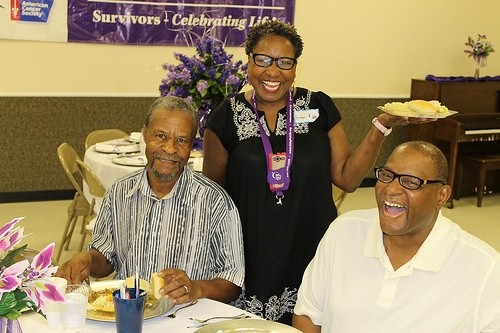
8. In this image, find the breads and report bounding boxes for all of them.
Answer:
[385,100,449,115]
[88,272,165,312]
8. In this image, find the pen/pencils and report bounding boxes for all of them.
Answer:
[115,266,147,300]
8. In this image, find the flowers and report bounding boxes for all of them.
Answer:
[0,217,70,333]
[158,33,249,151]
[464,34,495,62]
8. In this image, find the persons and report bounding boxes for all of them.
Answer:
[292,141,500,333]
[52,95,245,305]
[202,19,438,326]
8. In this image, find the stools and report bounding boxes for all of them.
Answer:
[454,148,500,207]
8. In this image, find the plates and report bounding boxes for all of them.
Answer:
[96,139,204,173]
[71,281,174,322]
[194,319,303,333]
[376,106,458,119]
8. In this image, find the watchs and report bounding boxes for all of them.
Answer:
[370,117,393,136]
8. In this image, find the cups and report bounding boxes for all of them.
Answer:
[59,283,88,331]
[112,287,146,333]
[41,277,67,325]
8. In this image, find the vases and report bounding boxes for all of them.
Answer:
[475,61,480,79]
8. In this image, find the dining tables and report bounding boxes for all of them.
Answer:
[82,136,203,231]
[16,278,264,333]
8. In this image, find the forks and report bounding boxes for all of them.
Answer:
[189,313,245,323]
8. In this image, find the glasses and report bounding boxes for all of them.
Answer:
[249,51,297,70]
[374,165,445,190]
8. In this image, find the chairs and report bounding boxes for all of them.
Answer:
[54,129,132,266]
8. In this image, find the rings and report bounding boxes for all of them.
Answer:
[183,285,188,293]
